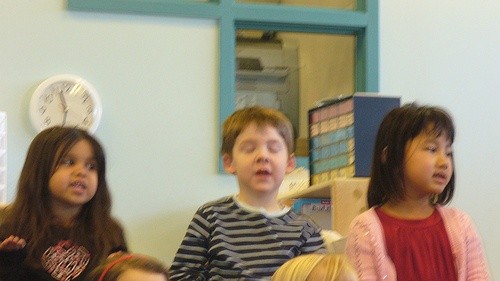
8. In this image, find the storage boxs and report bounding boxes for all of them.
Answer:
[307,93,401,187]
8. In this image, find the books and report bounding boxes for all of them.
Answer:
[292,197,332,217]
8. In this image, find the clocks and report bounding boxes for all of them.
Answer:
[29,75,102,136]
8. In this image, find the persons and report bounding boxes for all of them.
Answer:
[343,104,491,281]
[270,253,359,281]
[0,126,129,281]
[167,107,328,281]
[0,250,169,281]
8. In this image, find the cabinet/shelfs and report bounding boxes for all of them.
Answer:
[278,178,370,235]
